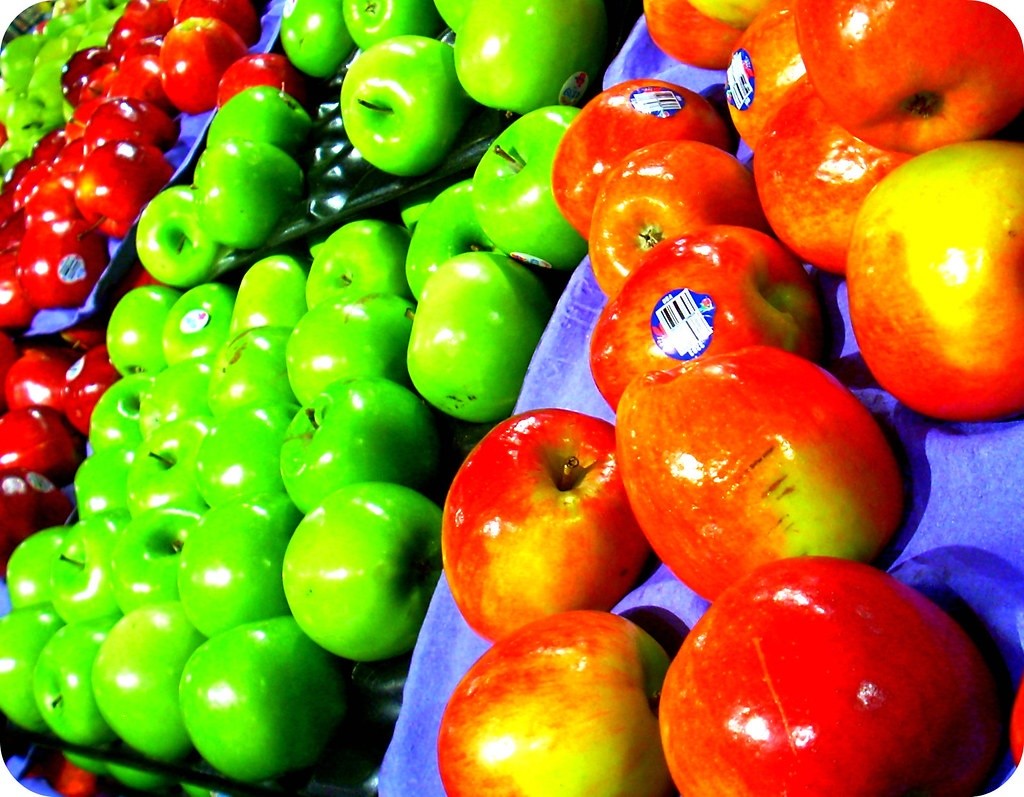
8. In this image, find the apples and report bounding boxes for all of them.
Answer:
[434,0,1024,797]
[0,0,603,783]
